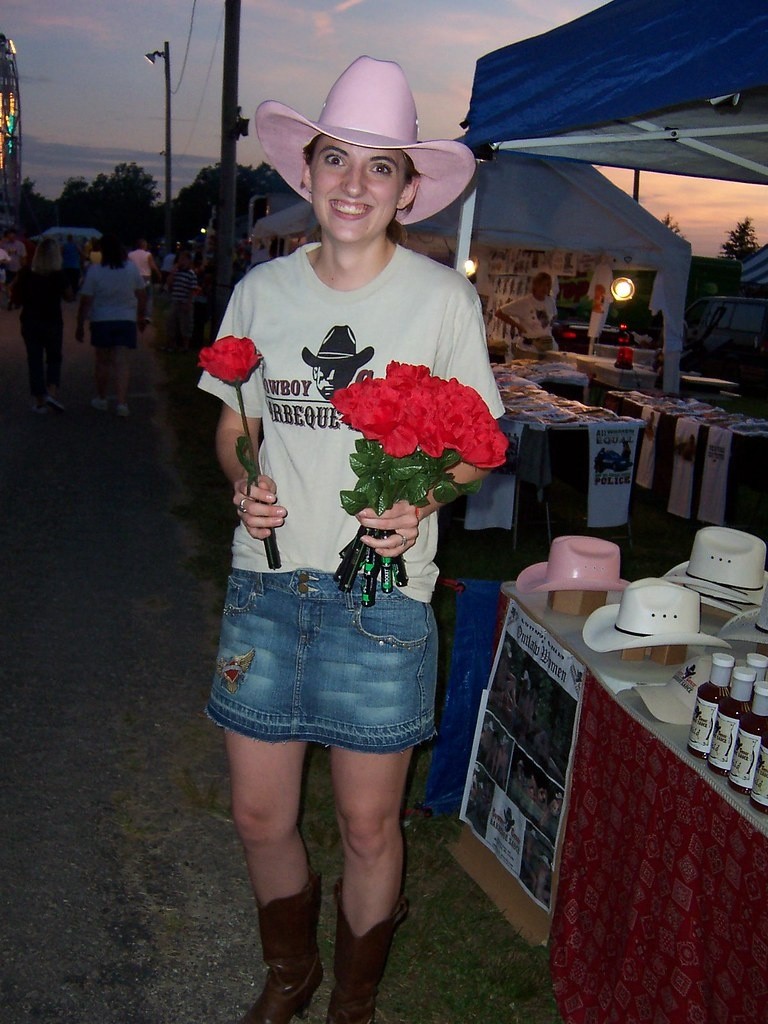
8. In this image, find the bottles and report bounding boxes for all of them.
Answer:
[686,653,768,815]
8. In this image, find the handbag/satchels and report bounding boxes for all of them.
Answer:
[530,336,553,351]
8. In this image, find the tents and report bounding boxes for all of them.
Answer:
[451,0,768,276]
[252,133,692,391]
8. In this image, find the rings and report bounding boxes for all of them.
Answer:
[240,498,246,512]
[397,533,406,546]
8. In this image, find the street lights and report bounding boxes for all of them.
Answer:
[145,41,173,233]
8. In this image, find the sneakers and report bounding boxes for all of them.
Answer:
[116,406,130,416]
[91,398,108,411]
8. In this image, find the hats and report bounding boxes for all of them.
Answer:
[631,655,711,725]
[582,577,732,653]
[716,571,768,645]
[256,55,476,225]
[515,535,630,594]
[663,525,768,613]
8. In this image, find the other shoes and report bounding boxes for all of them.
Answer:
[145,318,151,324]
[46,394,65,412]
[32,405,48,414]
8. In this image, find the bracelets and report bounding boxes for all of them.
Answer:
[415,507,419,517]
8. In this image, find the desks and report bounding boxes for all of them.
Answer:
[461,344,768,553]
[459,571,768,1024]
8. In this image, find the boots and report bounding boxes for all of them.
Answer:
[326,878,407,1024]
[237,867,323,1024]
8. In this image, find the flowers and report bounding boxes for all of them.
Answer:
[330,360,509,606]
[200,335,281,569]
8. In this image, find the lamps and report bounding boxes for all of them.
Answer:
[610,277,635,302]
[466,254,478,277]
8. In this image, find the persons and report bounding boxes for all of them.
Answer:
[0,230,33,310]
[129,239,162,325]
[6,240,74,416]
[61,235,102,303]
[158,234,217,352]
[495,274,559,350]
[77,241,147,417]
[196,54,504,1024]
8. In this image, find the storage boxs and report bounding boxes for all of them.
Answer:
[595,364,657,388]
[575,357,643,375]
